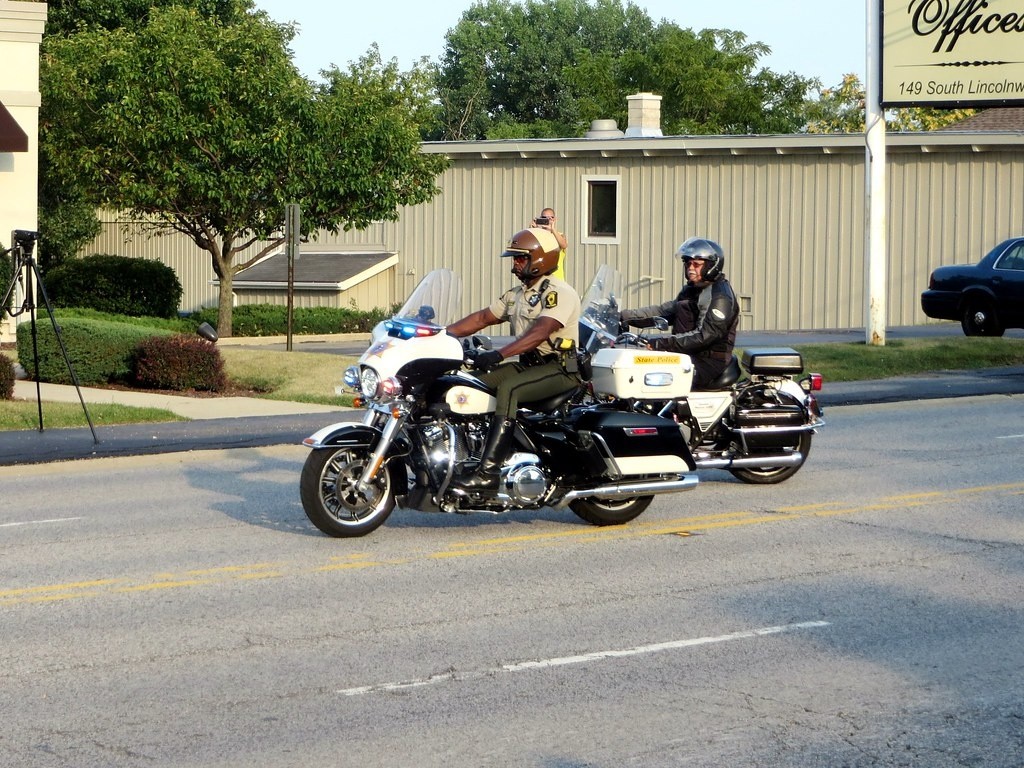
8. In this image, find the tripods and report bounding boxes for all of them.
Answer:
[0,241,100,444]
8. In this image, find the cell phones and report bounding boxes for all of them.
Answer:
[536,218,548,225]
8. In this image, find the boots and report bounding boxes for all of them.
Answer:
[445,416,515,498]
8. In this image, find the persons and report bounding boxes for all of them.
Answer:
[618,238,739,420]
[447,228,581,492]
[529,208,573,281]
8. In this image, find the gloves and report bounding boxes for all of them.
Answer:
[471,351,503,371]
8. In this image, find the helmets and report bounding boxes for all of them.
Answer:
[674,238,723,280]
[499,228,560,278]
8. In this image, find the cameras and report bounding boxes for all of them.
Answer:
[13,229,42,242]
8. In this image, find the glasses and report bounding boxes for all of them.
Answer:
[683,261,704,268]
[540,216,555,219]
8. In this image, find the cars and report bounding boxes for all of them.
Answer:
[922,238,1024,337]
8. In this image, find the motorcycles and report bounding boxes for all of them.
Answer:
[575,265,826,483]
[298,269,700,538]
